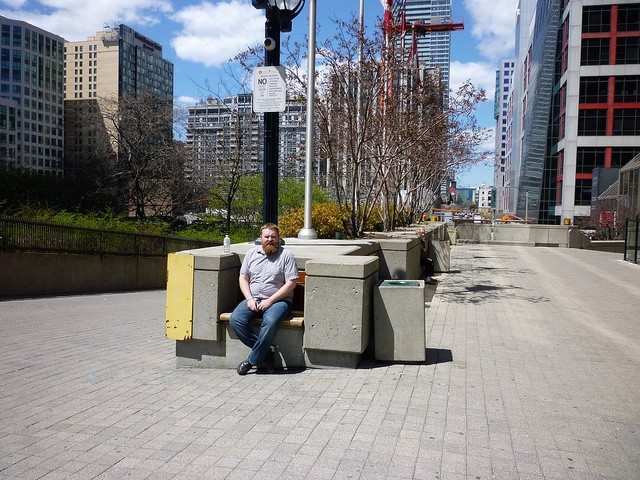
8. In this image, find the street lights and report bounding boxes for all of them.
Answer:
[252,0,307,224]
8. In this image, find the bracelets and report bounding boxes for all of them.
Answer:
[246,297,253,302]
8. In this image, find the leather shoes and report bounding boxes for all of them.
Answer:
[237,360,253,375]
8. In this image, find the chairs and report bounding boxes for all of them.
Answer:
[220,267,306,369]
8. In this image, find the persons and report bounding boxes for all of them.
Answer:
[229,222,300,375]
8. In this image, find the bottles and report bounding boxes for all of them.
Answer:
[223,235,230,253]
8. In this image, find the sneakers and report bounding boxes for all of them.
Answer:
[425,280,435,285]
[431,278,436,282]
